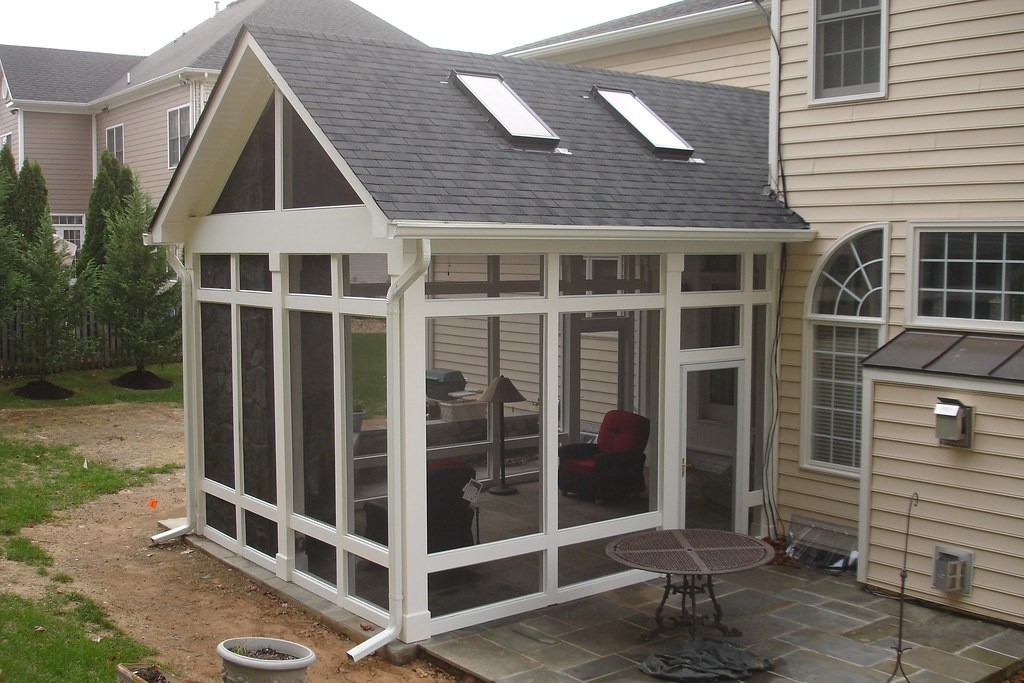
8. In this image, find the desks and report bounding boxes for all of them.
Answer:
[604,526,775,641]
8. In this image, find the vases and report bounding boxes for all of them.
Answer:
[115,662,182,683]
[215,636,316,683]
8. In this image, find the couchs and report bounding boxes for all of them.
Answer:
[559,408,650,505]
[363,459,477,555]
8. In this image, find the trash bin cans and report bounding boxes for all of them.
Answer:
[426,367,468,420]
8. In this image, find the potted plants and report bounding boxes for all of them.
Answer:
[352,398,365,431]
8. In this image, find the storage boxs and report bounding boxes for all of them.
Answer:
[427,367,466,399]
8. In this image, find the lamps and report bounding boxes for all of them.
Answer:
[477,374,527,496]
[11,108,18,114]
[100,106,109,114]
